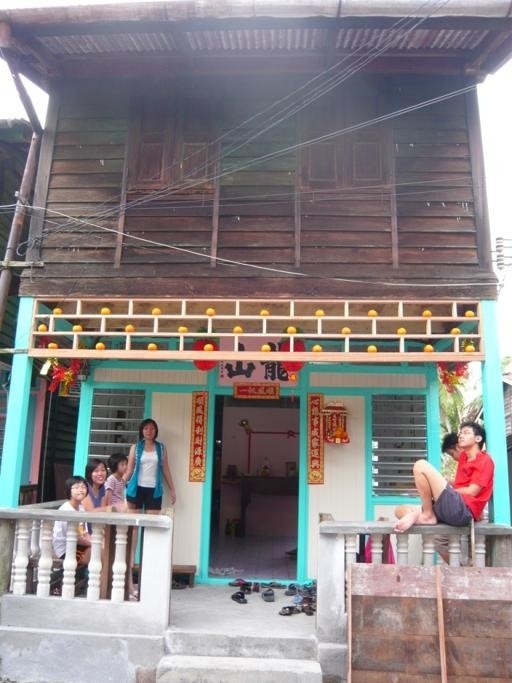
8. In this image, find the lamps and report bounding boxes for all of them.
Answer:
[491,234,512,271]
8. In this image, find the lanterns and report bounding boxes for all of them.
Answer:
[280,337,306,372]
[192,336,218,372]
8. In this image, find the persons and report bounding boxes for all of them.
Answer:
[83,459,113,551]
[54,475,110,597]
[122,418,177,563]
[105,453,140,602]
[435,431,470,567]
[392,420,492,532]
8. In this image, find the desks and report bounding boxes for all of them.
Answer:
[237,474,299,539]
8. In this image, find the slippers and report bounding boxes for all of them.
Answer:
[230,578,317,615]
